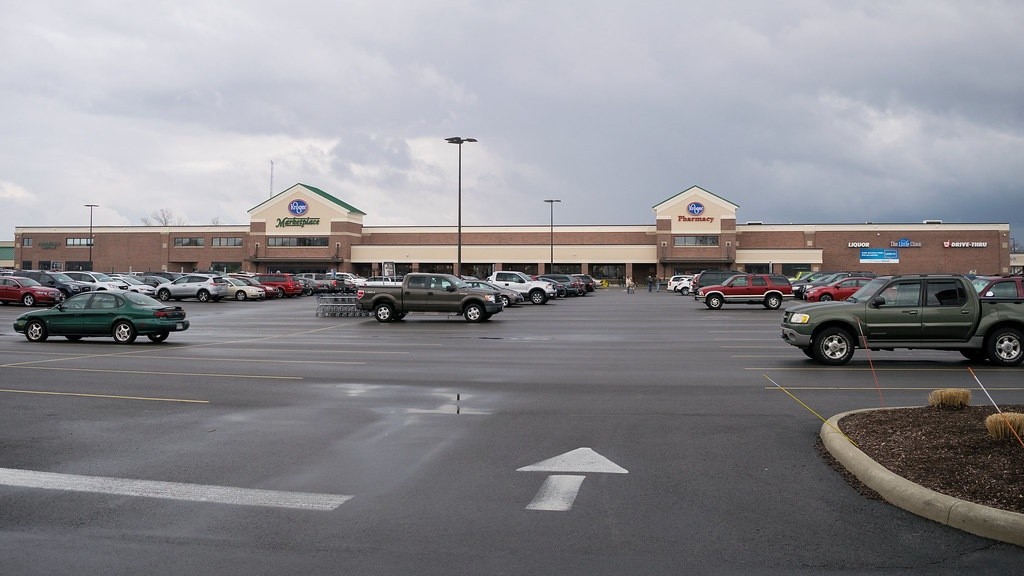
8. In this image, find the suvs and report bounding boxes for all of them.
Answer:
[0,269,120,307]
[666,270,796,310]
[462,271,604,306]
[296,272,405,295]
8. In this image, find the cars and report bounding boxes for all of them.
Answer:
[106,272,302,303]
[14,291,190,343]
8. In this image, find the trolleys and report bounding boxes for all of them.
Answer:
[314,290,375,317]
[628,282,635,294]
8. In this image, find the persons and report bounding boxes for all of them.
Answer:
[654,275,661,292]
[625,277,636,294]
[648,276,652,292]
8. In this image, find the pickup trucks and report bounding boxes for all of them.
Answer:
[781,271,1024,367]
[356,273,503,323]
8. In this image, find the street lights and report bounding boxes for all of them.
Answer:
[445,137,478,278]
[544,199,562,275]
[85,204,100,270]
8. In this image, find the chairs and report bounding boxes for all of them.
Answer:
[95,299,103,308]
[434,279,443,288]
